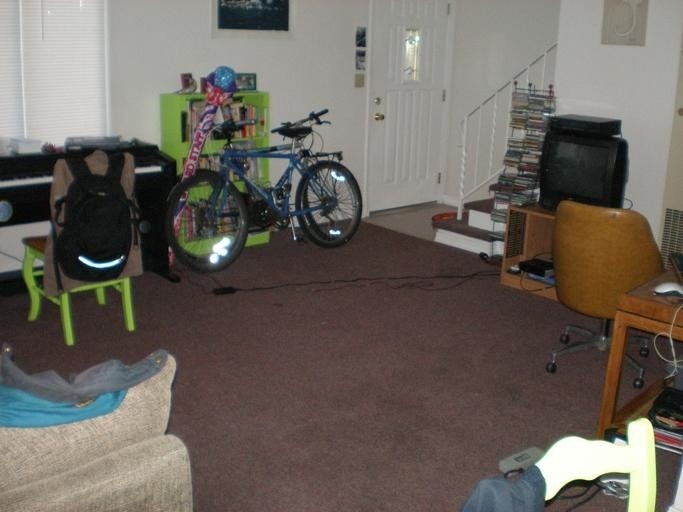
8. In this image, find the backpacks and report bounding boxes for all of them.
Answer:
[51,146,141,296]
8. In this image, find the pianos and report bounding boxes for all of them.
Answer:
[0,137,181,296]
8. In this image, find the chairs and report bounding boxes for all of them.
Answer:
[546,198,666,387]
[475,414,660,512]
[20,148,141,346]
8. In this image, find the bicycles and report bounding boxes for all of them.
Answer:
[163,109,362,274]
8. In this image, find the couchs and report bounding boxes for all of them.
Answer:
[0,346,194,511]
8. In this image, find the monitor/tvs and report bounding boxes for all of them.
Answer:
[539,131,629,211]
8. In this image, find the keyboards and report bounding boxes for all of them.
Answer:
[670,252,683,284]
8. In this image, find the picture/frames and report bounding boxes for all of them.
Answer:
[210,0,293,39]
[234,72,257,90]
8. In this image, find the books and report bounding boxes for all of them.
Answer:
[489,92,555,241]
[646,383,683,458]
[182,101,262,243]
[520,256,556,287]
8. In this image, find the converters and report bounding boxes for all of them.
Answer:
[213,287,235,295]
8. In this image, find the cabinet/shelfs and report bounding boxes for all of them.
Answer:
[157,89,272,254]
[487,79,556,241]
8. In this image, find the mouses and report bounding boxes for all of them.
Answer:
[655,282,683,297]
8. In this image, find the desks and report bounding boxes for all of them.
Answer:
[498,202,558,305]
[595,267,683,452]
